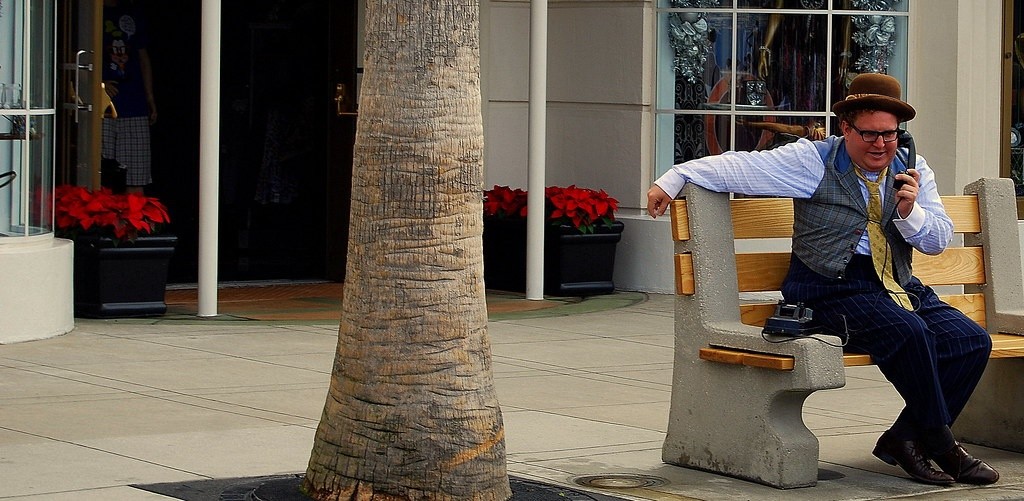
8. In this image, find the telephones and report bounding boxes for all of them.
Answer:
[894,130,916,192]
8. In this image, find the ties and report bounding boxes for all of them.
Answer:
[851,158,915,312]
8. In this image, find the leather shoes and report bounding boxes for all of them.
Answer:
[871,431,956,484]
[932,441,1000,485]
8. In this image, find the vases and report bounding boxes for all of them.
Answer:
[483,217,626,298]
[70,237,178,320]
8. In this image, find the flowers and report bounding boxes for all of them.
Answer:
[30,183,171,248]
[484,185,621,234]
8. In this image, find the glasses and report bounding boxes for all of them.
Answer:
[843,111,901,142]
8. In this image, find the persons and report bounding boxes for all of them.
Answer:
[647,73,1000,488]
[101,6,158,193]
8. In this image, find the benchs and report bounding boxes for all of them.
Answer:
[662,177,1024,490]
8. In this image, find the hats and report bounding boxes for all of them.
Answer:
[832,73,916,123]
